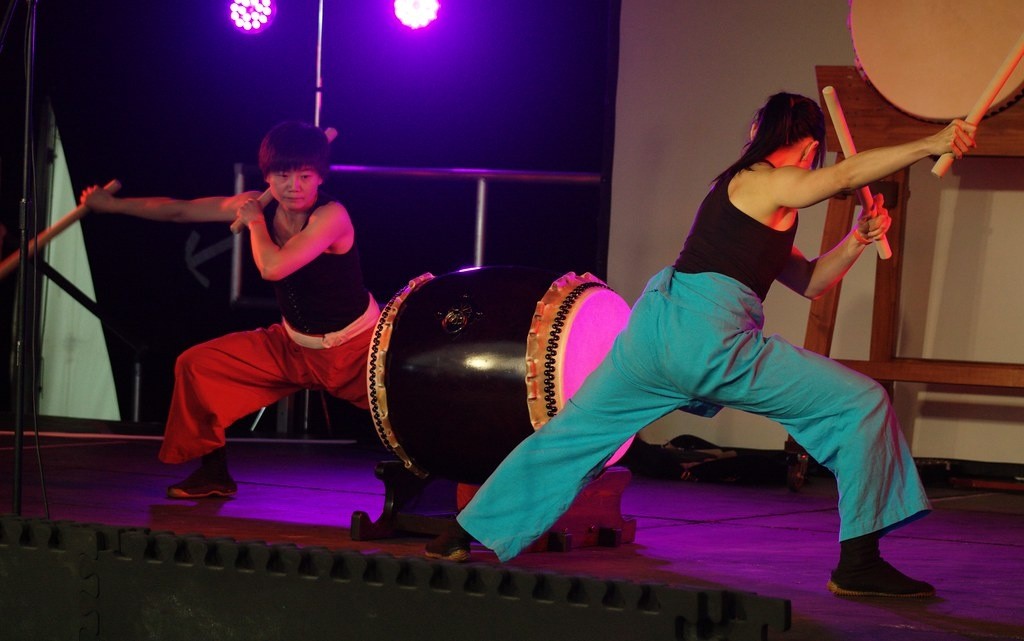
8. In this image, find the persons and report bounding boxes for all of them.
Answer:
[80,120,381,497]
[424,92,977,597]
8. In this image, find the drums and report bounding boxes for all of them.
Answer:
[847,0,1024,124]
[369,262,629,479]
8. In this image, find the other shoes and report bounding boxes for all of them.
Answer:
[425,528,471,561]
[829,557,935,599]
[167,468,238,498]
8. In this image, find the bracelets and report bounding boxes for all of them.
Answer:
[852,225,873,245]
